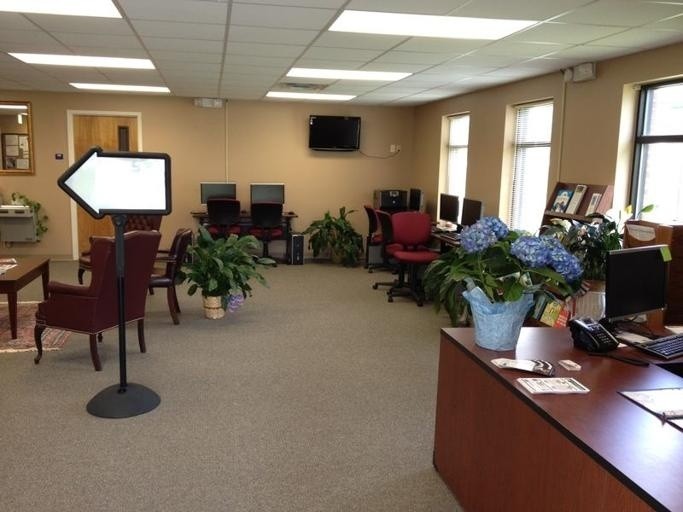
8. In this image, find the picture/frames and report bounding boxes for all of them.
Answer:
[2,133,29,170]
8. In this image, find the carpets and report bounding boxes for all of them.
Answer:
[0,302,69,351]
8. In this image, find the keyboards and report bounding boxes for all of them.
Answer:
[431,226,442,233]
[634,331,683,360]
[440,232,460,241]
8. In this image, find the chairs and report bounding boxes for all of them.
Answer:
[77,213,162,296]
[205,196,241,245]
[33,228,153,369]
[250,200,283,263]
[362,202,435,306]
[153,229,192,325]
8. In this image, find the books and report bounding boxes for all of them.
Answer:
[550,183,572,213]
[552,308,568,329]
[583,192,603,218]
[539,299,564,327]
[0,258,18,263]
[0,264,17,275]
[531,295,547,319]
[564,184,588,215]
[615,387,683,434]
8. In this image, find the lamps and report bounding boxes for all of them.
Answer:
[16,113,29,124]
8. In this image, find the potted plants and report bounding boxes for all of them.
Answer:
[304,208,364,263]
[533,204,656,319]
[182,225,273,319]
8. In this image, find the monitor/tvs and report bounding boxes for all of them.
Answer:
[462,198,481,226]
[201,182,236,204]
[250,183,285,205]
[605,244,669,324]
[309,115,361,151]
[409,188,423,211]
[440,194,458,223]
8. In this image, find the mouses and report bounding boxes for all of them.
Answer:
[288,211,294,214]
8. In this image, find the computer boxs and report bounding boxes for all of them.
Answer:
[373,190,406,211]
[288,231,304,265]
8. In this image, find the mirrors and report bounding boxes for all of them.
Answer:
[1,101,35,176]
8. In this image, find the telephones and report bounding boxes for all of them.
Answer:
[568,315,619,352]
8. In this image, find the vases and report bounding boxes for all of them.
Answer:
[463,287,537,350]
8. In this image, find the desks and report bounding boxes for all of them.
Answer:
[191,211,297,266]
[0,255,50,339]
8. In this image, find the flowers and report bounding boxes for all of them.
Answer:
[415,215,583,300]
